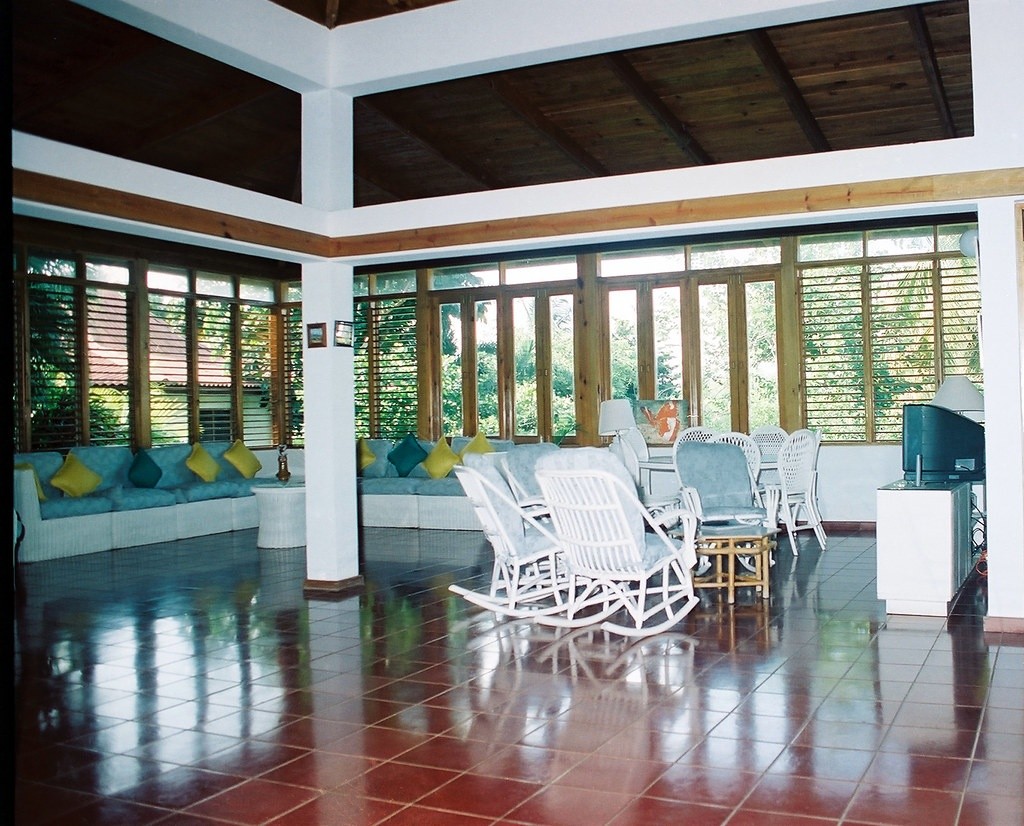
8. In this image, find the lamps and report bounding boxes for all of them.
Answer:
[598,399,636,442]
[276,445,290,482]
[928,375,984,415]
[334,321,354,348]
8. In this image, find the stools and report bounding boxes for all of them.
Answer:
[670,524,781,604]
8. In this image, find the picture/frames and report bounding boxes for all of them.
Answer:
[306,322,327,348]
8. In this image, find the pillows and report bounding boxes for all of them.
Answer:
[357,436,377,474]
[14,462,47,503]
[222,439,262,480]
[387,431,428,478]
[421,435,459,480]
[185,442,220,482]
[49,452,102,497]
[459,432,495,465]
[128,448,163,488]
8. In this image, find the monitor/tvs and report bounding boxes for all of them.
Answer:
[901,404,985,482]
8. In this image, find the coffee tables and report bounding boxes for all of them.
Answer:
[251,483,306,549]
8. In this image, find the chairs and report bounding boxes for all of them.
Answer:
[449,441,766,636]
[621,426,827,574]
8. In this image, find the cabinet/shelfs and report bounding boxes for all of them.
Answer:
[971,479,987,555]
[876,478,972,617]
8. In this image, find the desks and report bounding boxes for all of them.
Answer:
[642,496,678,511]
[639,454,778,470]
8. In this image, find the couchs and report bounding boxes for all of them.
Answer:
[14,441,287,563]
[357,439,513,532]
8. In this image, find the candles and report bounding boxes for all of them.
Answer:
[916,454,921,486]
